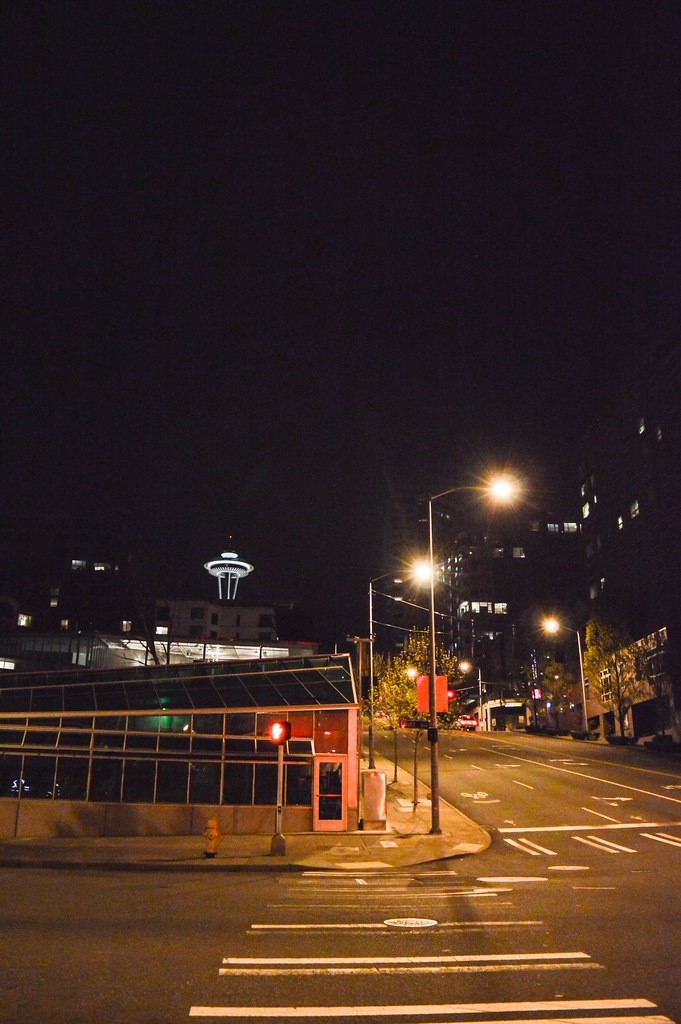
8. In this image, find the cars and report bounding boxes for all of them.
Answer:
[454,714,479,732]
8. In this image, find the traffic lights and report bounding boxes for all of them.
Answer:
[267,720,292,744]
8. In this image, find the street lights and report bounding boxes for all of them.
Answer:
[545,620,589,732]
[430,477,522,834]
[461,662,482,731]
[368,565,432,768]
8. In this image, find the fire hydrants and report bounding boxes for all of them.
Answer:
[203,815,221,857]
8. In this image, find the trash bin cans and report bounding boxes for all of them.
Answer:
[362,769,388,830]
